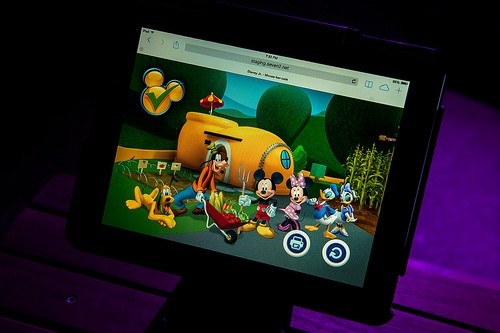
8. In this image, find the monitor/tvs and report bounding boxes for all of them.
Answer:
[66,3,446,325]
